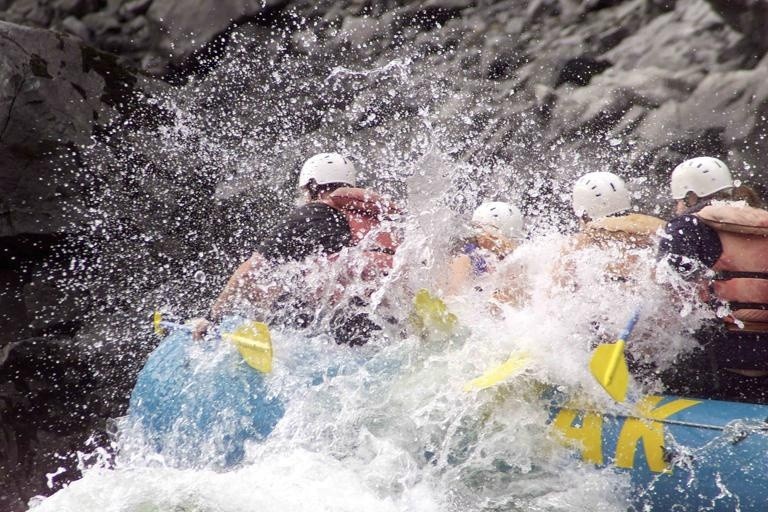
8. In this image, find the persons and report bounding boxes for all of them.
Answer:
[561,167,668,283]
[443,198,527,313]
[624,156,767,404]
[193,150,406,347]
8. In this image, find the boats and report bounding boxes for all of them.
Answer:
[130,318,767,512]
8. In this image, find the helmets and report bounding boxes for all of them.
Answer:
[573,172,632,219]
[299,153,356,188]
[670,157,732,201]
[472,202,523,239]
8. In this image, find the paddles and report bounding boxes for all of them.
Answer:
[152,308,273,376]
[412,285,460,334]
[467,353,531,391]
[588,310,641,405]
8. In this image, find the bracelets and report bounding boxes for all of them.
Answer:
[203,308,223,324]
